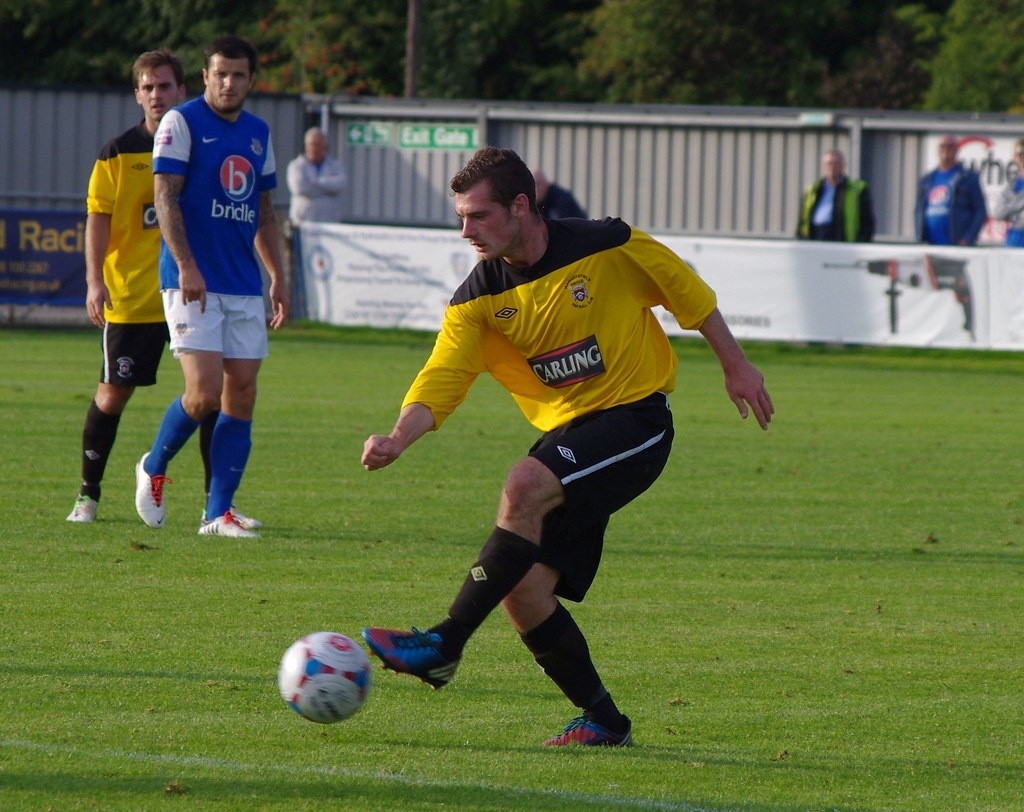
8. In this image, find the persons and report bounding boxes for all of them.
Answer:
[66,51,262,529]
[363,146,774,746]
[913,134,985,246]
[798,148,876,245]
[533,172,587,221]
[286,128,347,224]
[135,37,289,539]
[993,137,1024,248]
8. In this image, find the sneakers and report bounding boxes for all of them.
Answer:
[543,714,633,748]
[362,625,461,690]
[197,511,260,538]
[135,451,172,527]
[66,495,96,523]
[228,506,260,530]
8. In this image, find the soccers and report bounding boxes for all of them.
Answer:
[277,631,372,725]
